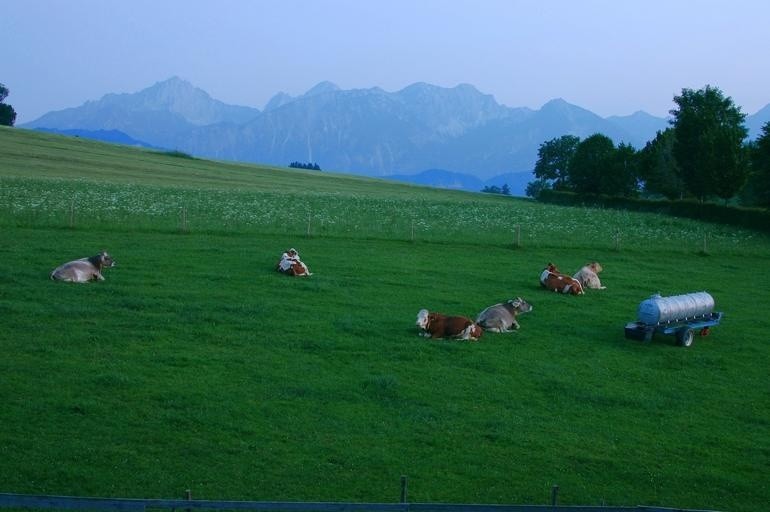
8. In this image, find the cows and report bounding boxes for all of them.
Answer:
[474,296,533,334]
[50,251,118,284]
[539,262,585,296]
[415,308,484,342]
[572,262,607,290]
[276,247,314,277]
